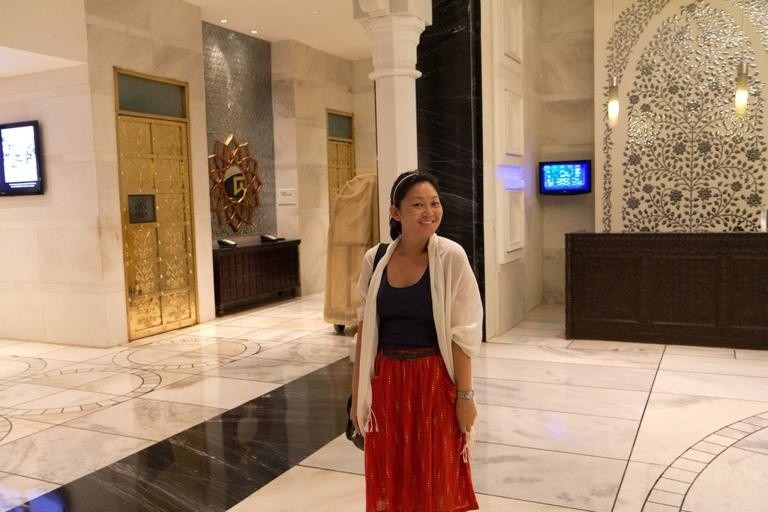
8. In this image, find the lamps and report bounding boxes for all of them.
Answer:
[606,1,621,126]
[735,0,750,116]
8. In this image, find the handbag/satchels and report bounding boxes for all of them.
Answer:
[346,394,364,451]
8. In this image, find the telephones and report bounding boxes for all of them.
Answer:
[261,235,285,243]
[218,239,237,248]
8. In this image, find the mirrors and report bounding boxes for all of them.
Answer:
[209,133,262,233]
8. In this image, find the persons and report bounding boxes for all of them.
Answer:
[351,172,485,512]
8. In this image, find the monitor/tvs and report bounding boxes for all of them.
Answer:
[0,119,44,195]
[538,159,592,195]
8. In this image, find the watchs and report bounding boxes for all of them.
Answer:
[454,390,475,400]
